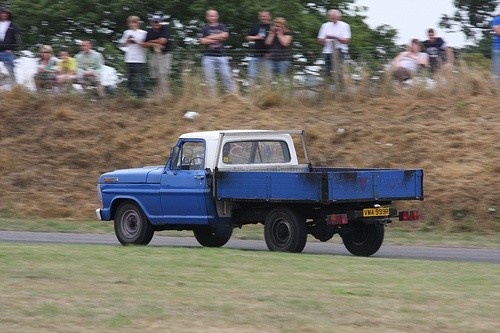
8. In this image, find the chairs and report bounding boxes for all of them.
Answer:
[84,65,117,95]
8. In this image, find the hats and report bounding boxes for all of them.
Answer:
[148,15,161,22]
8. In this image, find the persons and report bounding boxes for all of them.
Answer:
[488,14,500,81]
[245,11,293,81]
[391,27,454,86]
[119,14,173,99]
[33,40,104,99]
[199,9,238,96]
[0,8,22,83]
[318,9,351,77]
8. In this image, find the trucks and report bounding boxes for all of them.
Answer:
[94,128,425,257]
